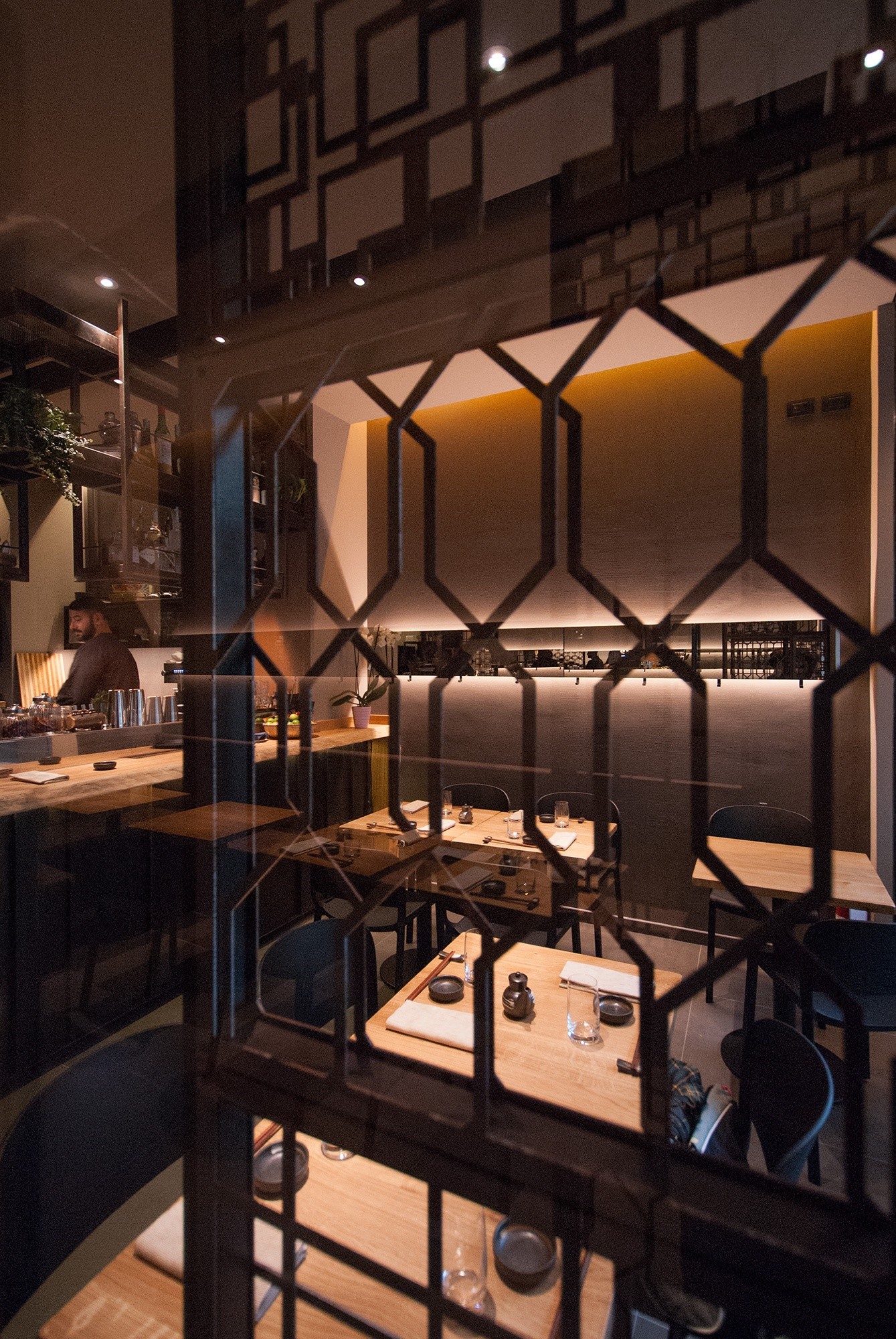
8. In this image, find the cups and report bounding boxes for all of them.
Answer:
[105,688,179,728]
[516,868,536,896]
[390,799,404,825]
[507,810,523,839]
[554,800,569,828]
[344,839,360,858]
[566,973,601,1046]
[436,1203,487,1308]
[442,791,453,816]
[463,928,481,988]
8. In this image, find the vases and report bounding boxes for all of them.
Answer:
[352,707,371,728]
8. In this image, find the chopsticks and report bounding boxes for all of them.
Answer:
[484,837,538,848]
[404,950,455,1001]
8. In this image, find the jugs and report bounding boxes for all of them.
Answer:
[502,971,535,1019]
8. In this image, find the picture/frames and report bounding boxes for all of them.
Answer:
[63,606,87,650]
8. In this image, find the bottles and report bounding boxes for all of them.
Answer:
[499,855,518,875]
[250,453,267,504]
[59,704,96,733]
[29,693,64,737]
[458,804,473,823]
[0,701,35,742]
[99,404,183,478]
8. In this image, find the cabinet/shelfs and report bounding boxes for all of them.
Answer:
[0,290,292,647]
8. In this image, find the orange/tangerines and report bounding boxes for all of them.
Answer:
[266,714,301,724]
[255,717,264,723]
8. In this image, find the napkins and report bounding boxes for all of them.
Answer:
[560,961,655,1004]
[402,800,428,814]
[418,819,455,834]
[134,1197,309,1320]
[546,831,576,851]
[503,809,524,823]
[384,999,484,1051]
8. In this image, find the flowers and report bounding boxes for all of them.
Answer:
[330,624,399,706]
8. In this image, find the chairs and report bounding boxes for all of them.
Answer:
[0,782,896,1339]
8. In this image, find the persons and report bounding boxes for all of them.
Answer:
[55,598,139,720]
[532,650,623,670]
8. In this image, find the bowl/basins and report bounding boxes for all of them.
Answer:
[538,814,555,823]
[250,1140,310,1192]
[482,880,506,896]
[320,843,340,855]
[593,995,634,1024]
[37,756,61,764]
[428,975,465,1001]
[491,1216,558,1284]
[409,821,417,829]
[263,723,317,738]
[93,761,116,770]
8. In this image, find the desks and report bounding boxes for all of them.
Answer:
[37,1117,619,1339]
[350,933,685,1137]
[451,811,625,962]
[690,834,896,1028]
[337,801,500,951]
[55,786,192,1012]
[128,801,307,996]
